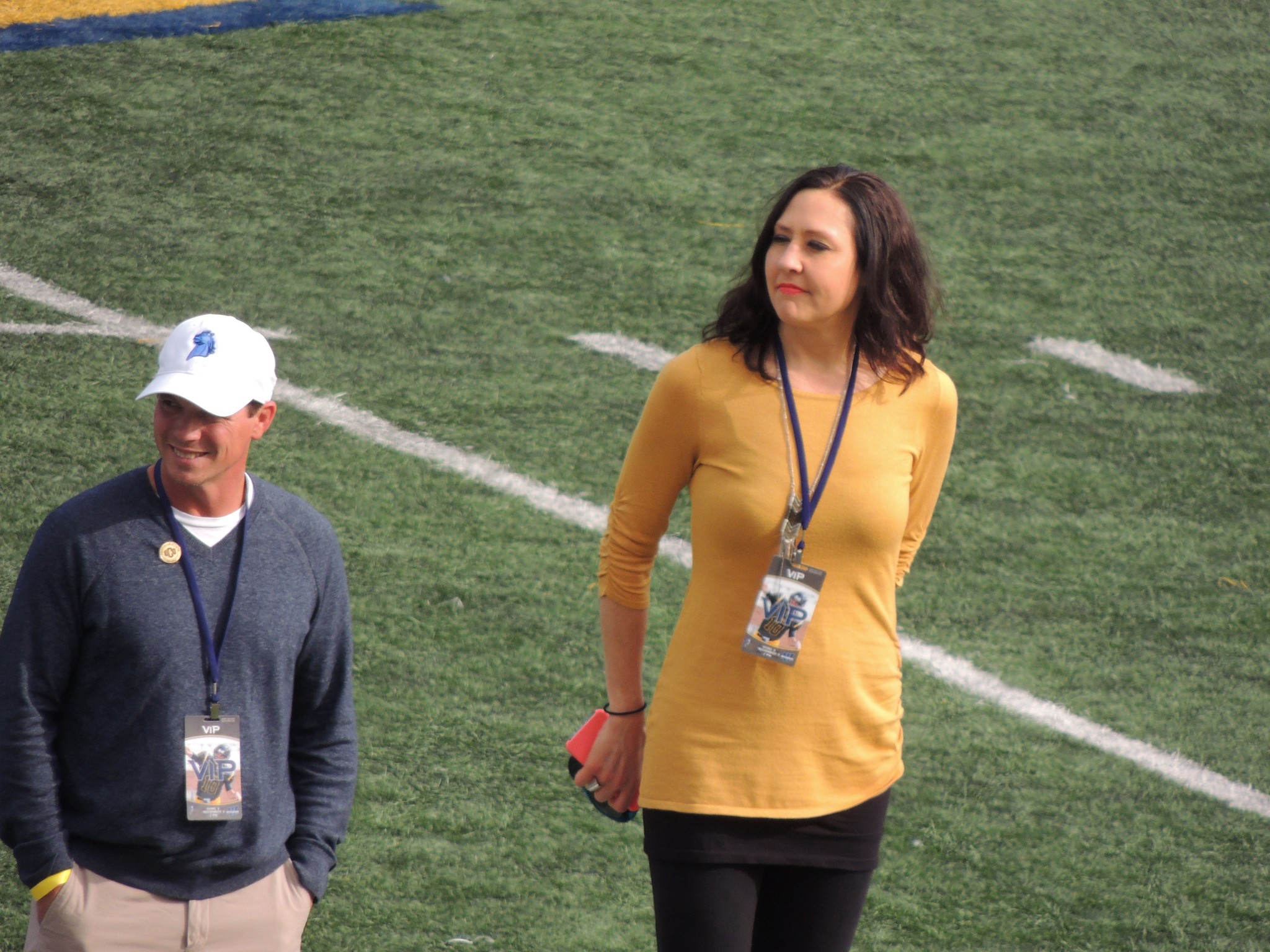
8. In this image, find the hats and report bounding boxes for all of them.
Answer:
[135,313,277,419]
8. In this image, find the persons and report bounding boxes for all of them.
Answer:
[0,314,358,952]
[574,164,959,952]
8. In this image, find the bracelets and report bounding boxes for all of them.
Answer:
[603,701,647,715]
[30,868,71,903]
